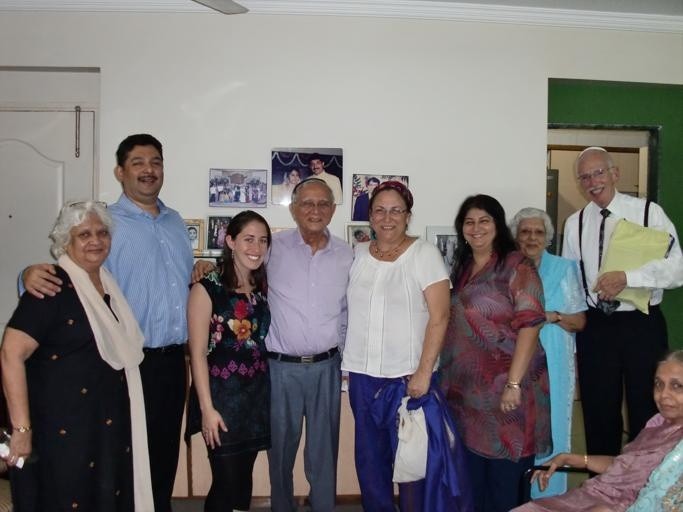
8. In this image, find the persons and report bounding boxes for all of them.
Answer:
[509,348,683,512]
[0,200,155,512]
[18,133,194,512]
[508,207,589,500]
[438,194,554,512]
[187,210,272,510]
[560,146,683,480]
[340,181,454,511]
[301,152,342,204]
[352,177,380,221]
[209,176,267,205]
[191,177,354,511]
[277,168,302,204]
[188,220,227,250]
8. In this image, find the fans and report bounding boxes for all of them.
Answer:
[192,0,249,15]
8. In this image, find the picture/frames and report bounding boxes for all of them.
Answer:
[426,225,462,277]
[343,223,374,250]
[184,219,204,257]
[204,212,235,258]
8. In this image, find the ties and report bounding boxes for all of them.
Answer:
[596,209,610,273]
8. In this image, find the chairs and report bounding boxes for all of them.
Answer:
[504,467,600,512]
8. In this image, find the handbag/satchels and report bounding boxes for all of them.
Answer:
[391,392,458,484]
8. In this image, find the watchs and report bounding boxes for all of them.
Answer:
[12,426,32,433]
[551,311,562,324]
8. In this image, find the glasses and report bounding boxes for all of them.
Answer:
[574,166,618,182]
[371,206,409,217]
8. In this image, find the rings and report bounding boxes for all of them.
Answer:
[203,432,208,437]
[511,404,517,410]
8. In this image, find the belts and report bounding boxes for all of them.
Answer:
[138,342,185,353]
[267,346,340,364]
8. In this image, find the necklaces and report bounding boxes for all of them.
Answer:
[373,237,406,257]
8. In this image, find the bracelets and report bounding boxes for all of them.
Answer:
[504,381,522,390]
[584,454,588,469]
